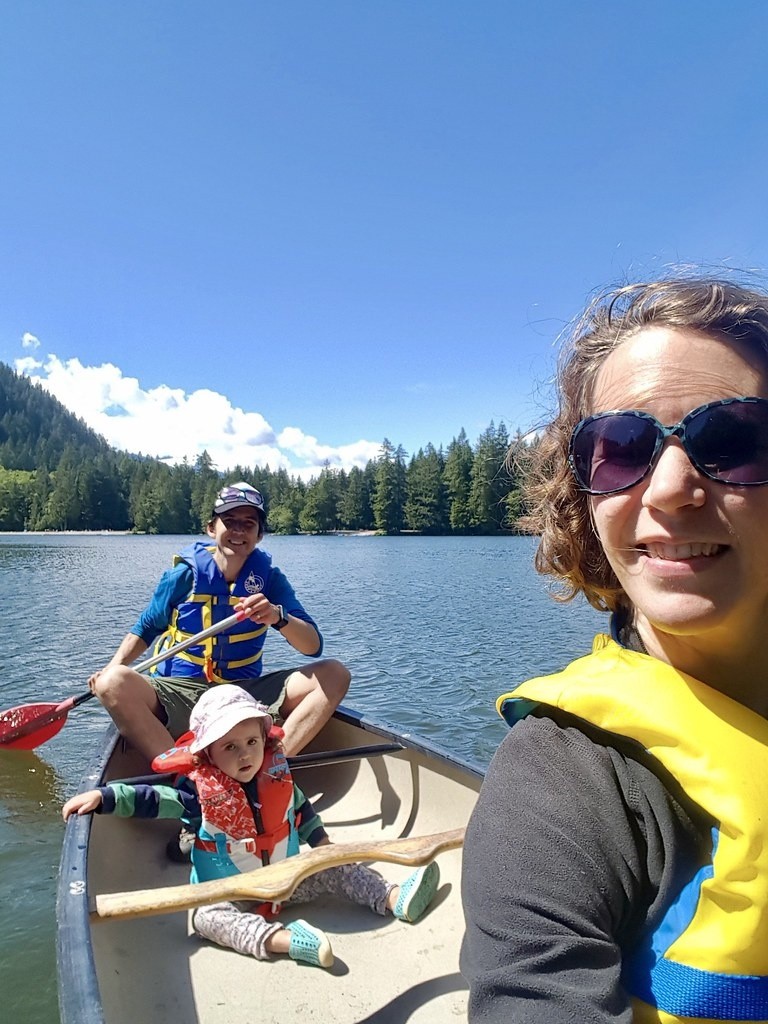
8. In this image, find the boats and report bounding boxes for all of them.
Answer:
[57,667,485,1024]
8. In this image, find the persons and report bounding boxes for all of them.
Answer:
[84,480,354,863]
[455,274,768,1023]
[62,682,441,968]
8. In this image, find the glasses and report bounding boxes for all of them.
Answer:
[215,487,264,505]
[568,397,768,496]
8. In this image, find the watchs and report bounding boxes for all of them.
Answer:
[271,603,289,631]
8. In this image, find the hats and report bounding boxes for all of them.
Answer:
[210,482,268,522]
[188,681,273,756]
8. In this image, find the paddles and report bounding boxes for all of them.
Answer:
[0,608,249,751]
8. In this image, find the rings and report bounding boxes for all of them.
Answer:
[255,612,261,620]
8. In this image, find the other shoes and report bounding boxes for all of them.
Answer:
[167,819,197,861]
[285,919,333,968]
[394,859,439,922]
